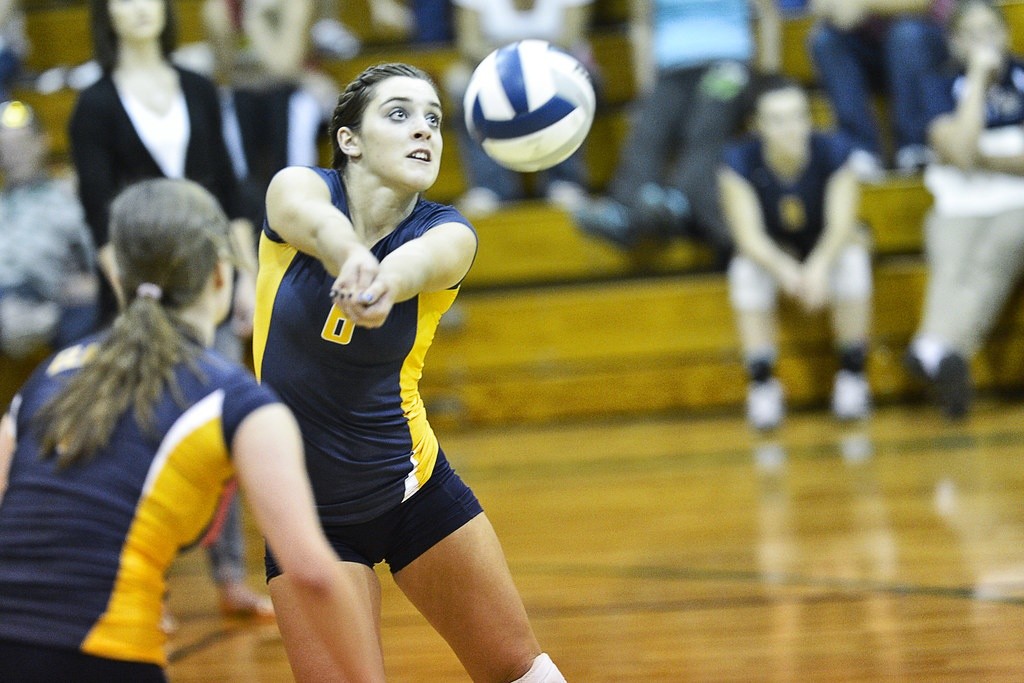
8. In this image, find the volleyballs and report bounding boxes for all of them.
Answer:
[461,37,599,177]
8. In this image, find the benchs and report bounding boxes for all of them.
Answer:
[2,0,1024,432]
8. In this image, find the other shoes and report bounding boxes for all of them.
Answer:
[851,147,884,187]
[217,580,276,618]
[639,181,689,233]
[897,144,931,185]
[748,376,783,428]
[159,599,179,633]
[831,370,870,420]
[906,350,970,419]
[552,178,636,250]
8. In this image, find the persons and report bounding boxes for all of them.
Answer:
[443,2,602,221]
[0,0,332,635]
[904,1,1024,415]
[0,174,389,683]
[548,0,783,267]
[802,0,961,179]
[714,74,873,428]
[253,65,571,683]
